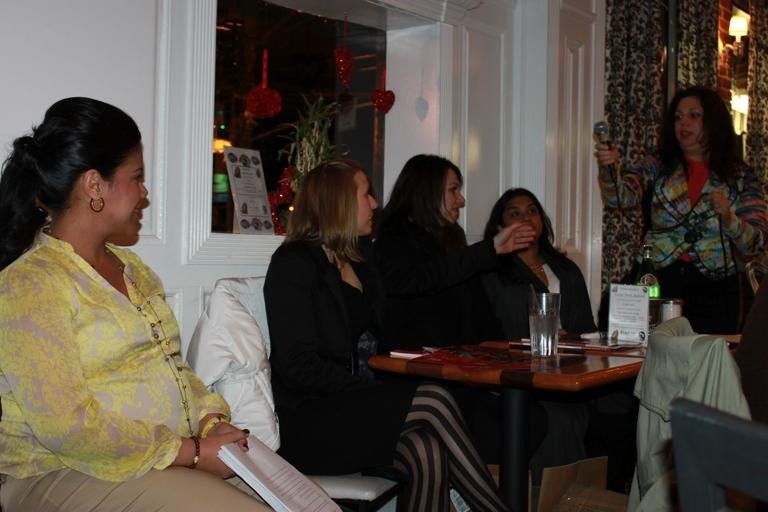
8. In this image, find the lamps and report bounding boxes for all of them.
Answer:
[724,16,748,57]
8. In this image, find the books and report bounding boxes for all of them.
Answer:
[508,340,623,350]
[521,335,641,348]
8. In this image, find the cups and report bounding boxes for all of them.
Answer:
[528,292,562,356]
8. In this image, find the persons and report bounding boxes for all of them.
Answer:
[727,272,768,425]
[262,160,510,511]
[475,188,609,510]
[363,153,552,511]
[593,86,767,335]
[0,93,280,512]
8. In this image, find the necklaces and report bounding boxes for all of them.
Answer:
[528,262,547,272]
[90,249,194,436]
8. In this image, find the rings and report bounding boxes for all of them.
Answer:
[717,206,721,214]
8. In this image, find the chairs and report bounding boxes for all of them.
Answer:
[669,395,768,511]
[213,275,402,511]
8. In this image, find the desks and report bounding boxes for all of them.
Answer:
[366,326,740,512]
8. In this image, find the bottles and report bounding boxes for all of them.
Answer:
[635,244,661,328]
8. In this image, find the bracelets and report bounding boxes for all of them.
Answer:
[187,436,199,470]
[199,417,228,439]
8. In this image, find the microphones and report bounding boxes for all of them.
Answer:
[594,119,620,189]
